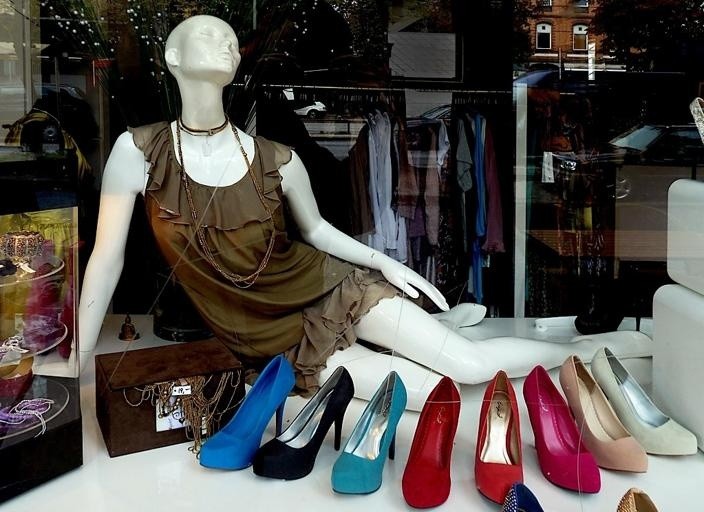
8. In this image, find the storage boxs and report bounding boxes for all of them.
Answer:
[94,338,245,458]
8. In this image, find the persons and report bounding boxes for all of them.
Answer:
[34,11,655,415]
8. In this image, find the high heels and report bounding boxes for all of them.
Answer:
[524,346,698,493]
[332,372,406,493]
[402,376,460,507]
[477,370,524,504]
[575,281,643,336]
[200,355,296,470]
[254,367,353,480]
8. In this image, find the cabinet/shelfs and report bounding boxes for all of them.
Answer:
[1,144,84,506]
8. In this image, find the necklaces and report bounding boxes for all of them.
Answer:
[176,113,277,289]
[0,397,55,439]
[177,111,230,137]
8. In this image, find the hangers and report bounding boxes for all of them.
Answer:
[357,86,397,122]
[448,86,511,124]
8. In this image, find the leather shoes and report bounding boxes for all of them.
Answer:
[616,488,658,511]
[503,482,545,512]
